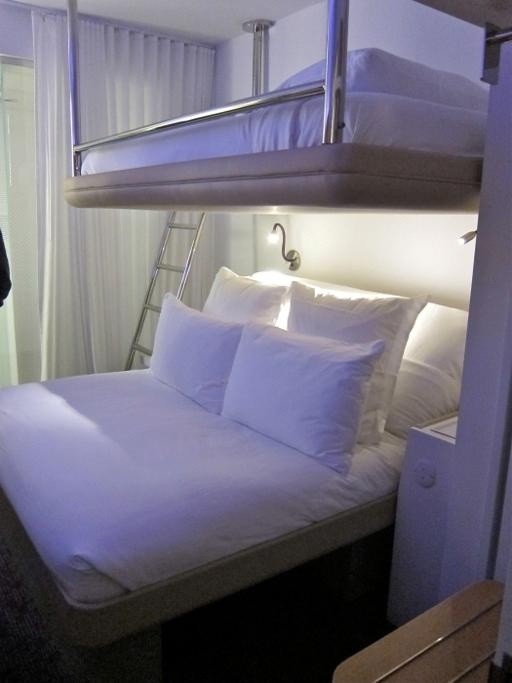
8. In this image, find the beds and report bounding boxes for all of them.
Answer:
[0,366,472,680]
[58,0,493,221]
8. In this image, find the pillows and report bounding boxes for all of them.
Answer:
[271,45,489,108]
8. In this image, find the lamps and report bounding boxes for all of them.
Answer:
[267,222,303,272]
[452,224,477,247]
